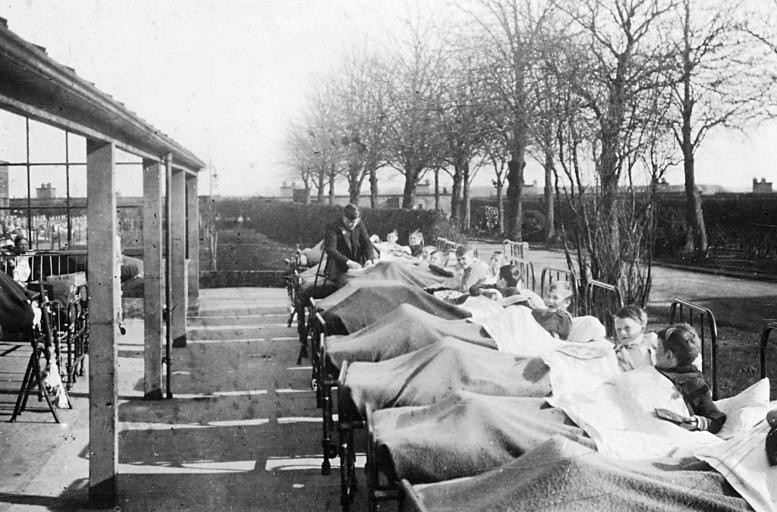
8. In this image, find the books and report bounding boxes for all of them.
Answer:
[655,408,686,425]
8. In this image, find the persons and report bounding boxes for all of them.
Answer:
[487,250,509,272]
[6,232,43,291]
[453,244,496,292]
[765,409,777,467]
[531,279,574,341]
[397,226,425,256]
[365,231,380,245]
[428,248,446,266]
[613,301,654,372]
[0,268,73,345]
[654,321,728,433]
[385,229,400,252]
[467,264,524,303]
[321,200,376,282]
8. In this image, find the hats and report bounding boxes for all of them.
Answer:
[344,204,359,219]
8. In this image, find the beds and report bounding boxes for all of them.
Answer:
[286,236,777,511]
